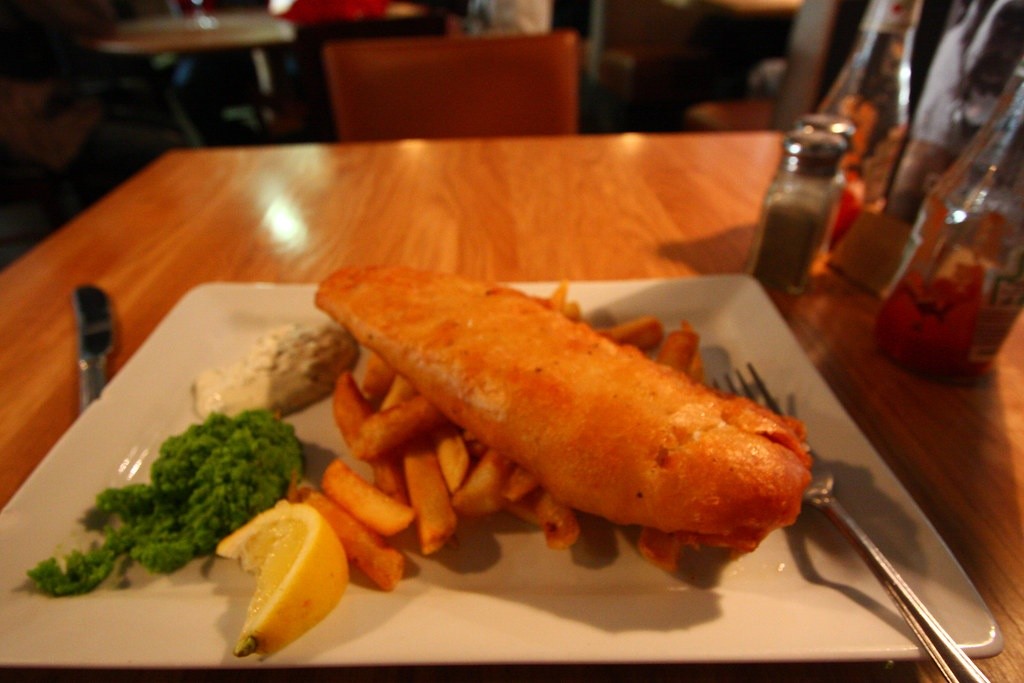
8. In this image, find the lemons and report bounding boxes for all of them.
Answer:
[217,500,348,658]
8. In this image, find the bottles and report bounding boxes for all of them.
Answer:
[741,113,855,292]
[812,1,924,212]
[872,56,1024,382]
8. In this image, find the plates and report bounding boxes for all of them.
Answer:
[1,274,1005,668]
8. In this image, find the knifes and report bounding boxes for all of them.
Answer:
[72,282,114,415]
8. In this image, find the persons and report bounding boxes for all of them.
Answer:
[1,1,308,230]
[622,1,805,133]
[465,1,603,136]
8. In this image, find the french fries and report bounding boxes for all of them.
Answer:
[294,278,703,579]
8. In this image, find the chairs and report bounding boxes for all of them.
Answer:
[321,29,578,141]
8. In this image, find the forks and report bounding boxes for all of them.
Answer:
[709,362,993,682]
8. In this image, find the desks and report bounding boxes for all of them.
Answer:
[0,134,1024,683]
[68,3,428,149]
